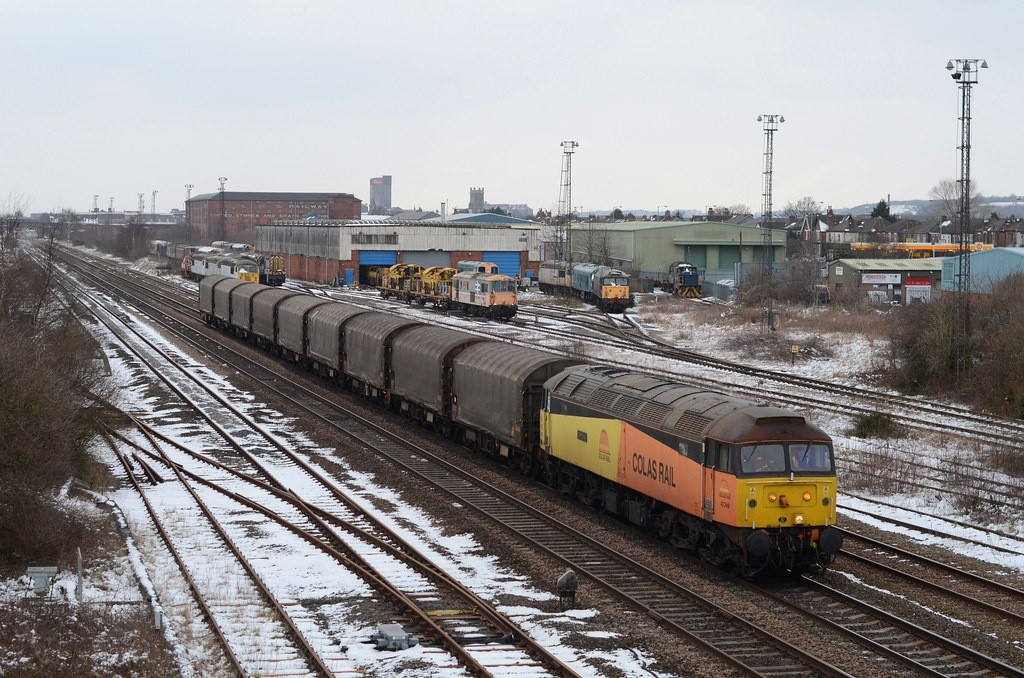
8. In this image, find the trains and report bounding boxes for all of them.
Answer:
[367,259,519,322]
[144,240,286,286]
[198,273,843,591]
[539,259,633,312]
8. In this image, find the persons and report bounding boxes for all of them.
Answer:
[515,274,520,286]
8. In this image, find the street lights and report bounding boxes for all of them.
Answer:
[184,184,195,244]
[151,190,159,222]
[753,114,785,337]
[553,140,580,306]
[939,59,989,404]
[217,177,228,241]
[137,193,145,224]
